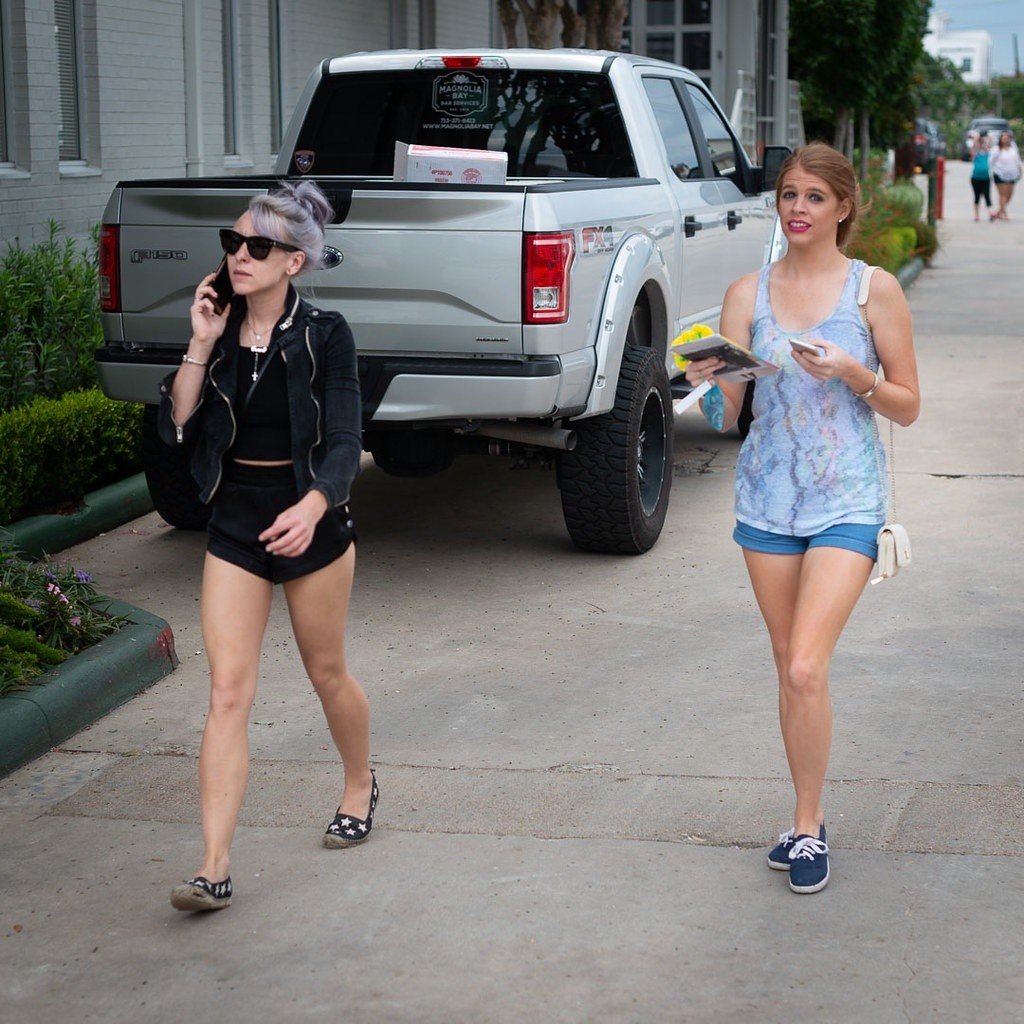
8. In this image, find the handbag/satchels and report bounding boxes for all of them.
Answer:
[871,523,911,585]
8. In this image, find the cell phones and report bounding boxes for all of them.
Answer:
[789,339,822,357]
[207,255,233,315]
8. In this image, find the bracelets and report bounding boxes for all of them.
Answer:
[853,371,880,399]
[180,353,209,366]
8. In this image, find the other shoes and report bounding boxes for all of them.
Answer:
[324,771,380,849]
[170,876,233,911]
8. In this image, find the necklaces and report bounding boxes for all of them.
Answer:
[245,321,276,383]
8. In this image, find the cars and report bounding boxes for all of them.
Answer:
[914,119,947,174]
[961,117,1014,162]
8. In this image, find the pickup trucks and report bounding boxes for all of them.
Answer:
[92,46,794,556]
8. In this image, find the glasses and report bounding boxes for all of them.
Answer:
[219,228,302,260]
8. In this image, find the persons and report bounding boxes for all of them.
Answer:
[966,128,1021,221]
[155,182,380,911]
[684,142,921,894]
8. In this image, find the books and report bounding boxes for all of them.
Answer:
[670,333,779,386]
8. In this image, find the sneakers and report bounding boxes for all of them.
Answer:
[788,819,830,894]
[767,827,795,870]
